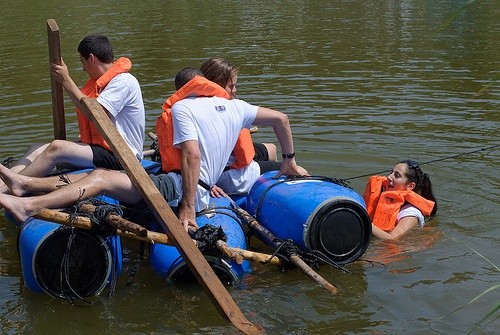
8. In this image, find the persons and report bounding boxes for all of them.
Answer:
[0,34,314,234]
[370,160,438,242]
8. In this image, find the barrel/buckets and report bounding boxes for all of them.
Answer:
[149,195,247,288]
[246,170,372,265]
[18,168,122,300]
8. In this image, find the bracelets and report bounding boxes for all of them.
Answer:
[282,151,296,159]
